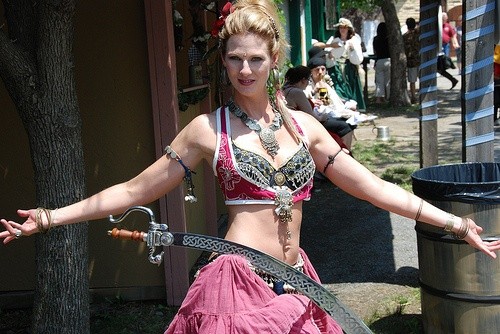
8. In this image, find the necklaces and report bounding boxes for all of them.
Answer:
[228,95,284,161]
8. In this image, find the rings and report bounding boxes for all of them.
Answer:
[14,230,22,239]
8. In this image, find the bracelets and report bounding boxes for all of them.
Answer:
[414,198,424,221]
[444,213,455,232]
[456,216,470,239]
[36,207,58,233]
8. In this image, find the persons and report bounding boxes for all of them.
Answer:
[303,46,358,150]
[453,15,462,75]
[373,22,391,105]
[0,0,500,334]
[442,12,460,56]
[402,17,420,104]
[324,18,366,112]
[437,55,459,90]
[280,65,354,158]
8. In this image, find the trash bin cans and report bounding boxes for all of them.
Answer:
[410,161,500,334]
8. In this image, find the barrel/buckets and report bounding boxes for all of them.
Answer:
[377,126,389,141]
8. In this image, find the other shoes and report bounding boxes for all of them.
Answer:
[413,98,418,103]
[375,99,382,104]
[386,99,390,104]
[452,79,458,88]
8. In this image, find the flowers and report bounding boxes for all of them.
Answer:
[211,2,232,39]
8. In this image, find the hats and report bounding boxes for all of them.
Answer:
[332,18,355,31]
[308,46,330,59]
[308,58,327,68]
